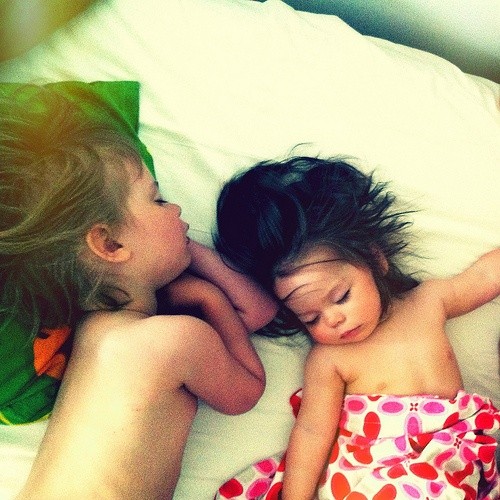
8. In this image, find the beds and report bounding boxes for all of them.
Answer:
[0,1,500,500]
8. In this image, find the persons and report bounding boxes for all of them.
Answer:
[0,81,279,499]
[210,142,500,499]
